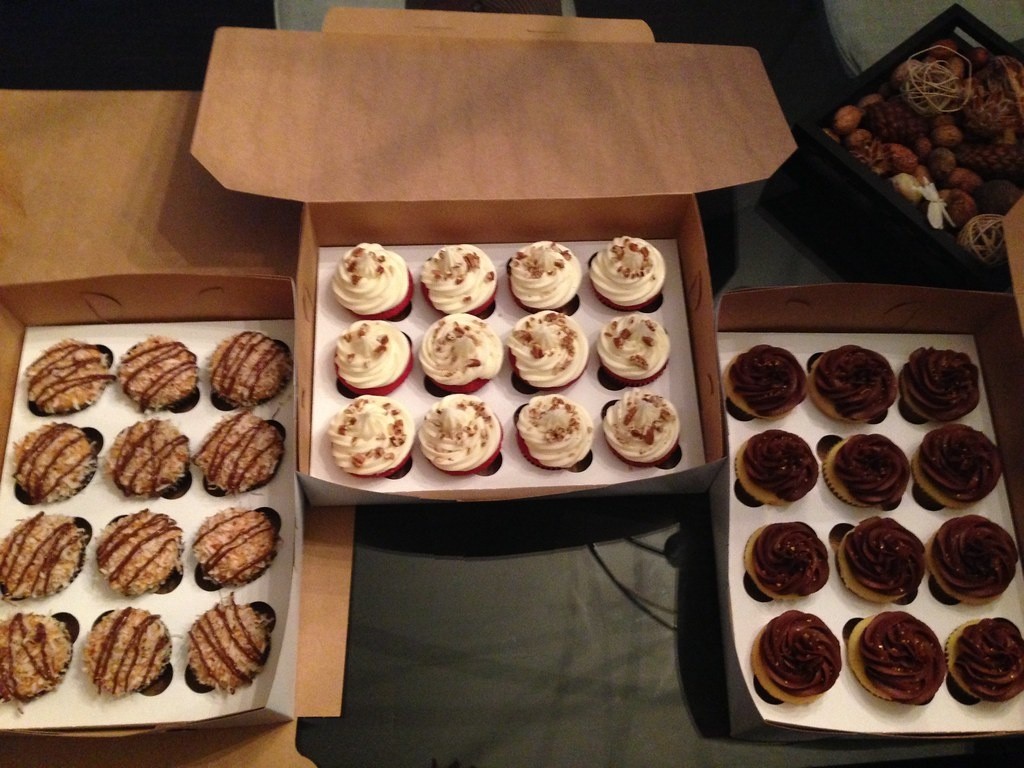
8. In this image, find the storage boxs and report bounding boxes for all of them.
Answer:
[0,4,1024,742]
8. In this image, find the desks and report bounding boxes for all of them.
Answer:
[0,89,1024,768]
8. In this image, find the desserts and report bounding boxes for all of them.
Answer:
[722,344,1024,705]
[327,237,682,479]
[0,331,292,702]
